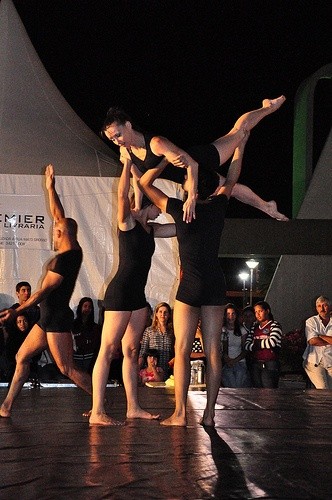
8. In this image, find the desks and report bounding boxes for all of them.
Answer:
[145,382,206,390]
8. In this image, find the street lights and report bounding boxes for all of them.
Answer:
[238,269,249,309]
[243,256,261,310]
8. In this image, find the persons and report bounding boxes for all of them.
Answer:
[0,163,92,417]
[0,296,283,387]
[302,294,332,389]
[135,128,251,427]
[87,156,176,426]
[7,280,41,328]
[98,96,289,224]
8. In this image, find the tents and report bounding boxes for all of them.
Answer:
[261,132,331,336]
[0,0,182,322]
